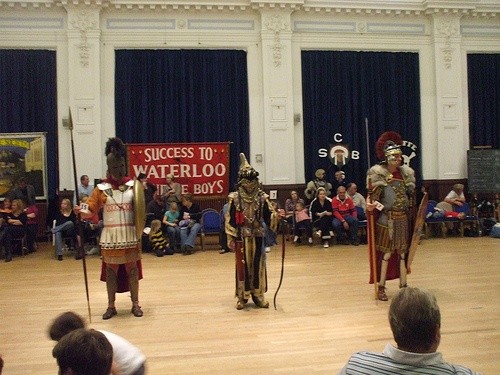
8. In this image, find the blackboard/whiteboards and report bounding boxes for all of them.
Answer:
[467,149,499,194]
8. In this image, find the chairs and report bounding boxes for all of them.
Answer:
[196,209,226,252]
[284,198,500,242]
[10,218,98,259]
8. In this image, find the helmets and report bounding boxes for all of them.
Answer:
[375,131,404,167]
[105,138,129,179]
[238,152,259,178]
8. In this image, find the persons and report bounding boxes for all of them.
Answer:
[224,167,286,310]
[74,138,145,320]
[0,177,39,262]
[53,328,113,375]
[341,287,473,375]
[219,202,280,254]
[50,311,147,375]
[420,183,472,236]
[366,132,416,301]
[286,169,366,248]
[45,174,201,261]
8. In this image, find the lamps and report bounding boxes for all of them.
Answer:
[61,117,69,130]
[295,113,301,122]
[256,154,262,161]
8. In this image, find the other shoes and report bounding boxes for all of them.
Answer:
[323,240,329,248]
[308,237,313,244]
[377,285,388,301]
[352,240,359,246]
[400,285,407,289]
[180,245,192,255]
[57,254,63,261]
[5,254,12,262]
[220,249,226,254]
[102,307,117,320]
[131,305,143,317]
[265,247,271,253]
[75,252,82,260]
[316,229,322,238]
[252,294,270,308]
[337,240,349,245]
[293,235,299,242]
[236,298,248,310]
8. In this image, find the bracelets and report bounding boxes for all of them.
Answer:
[342,221,346,223]
